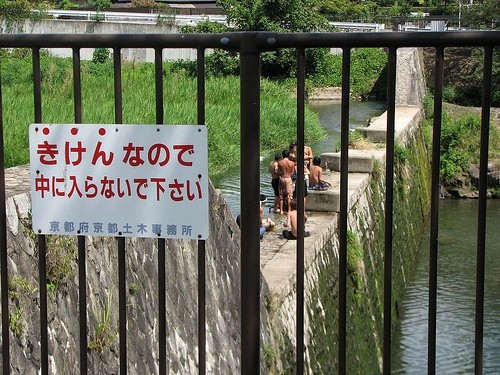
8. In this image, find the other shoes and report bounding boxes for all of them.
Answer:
[274,208,280,212]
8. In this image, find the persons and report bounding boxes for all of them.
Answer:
[236,194,275,239]
[283,198,308,238]
[269,142,331,214]
